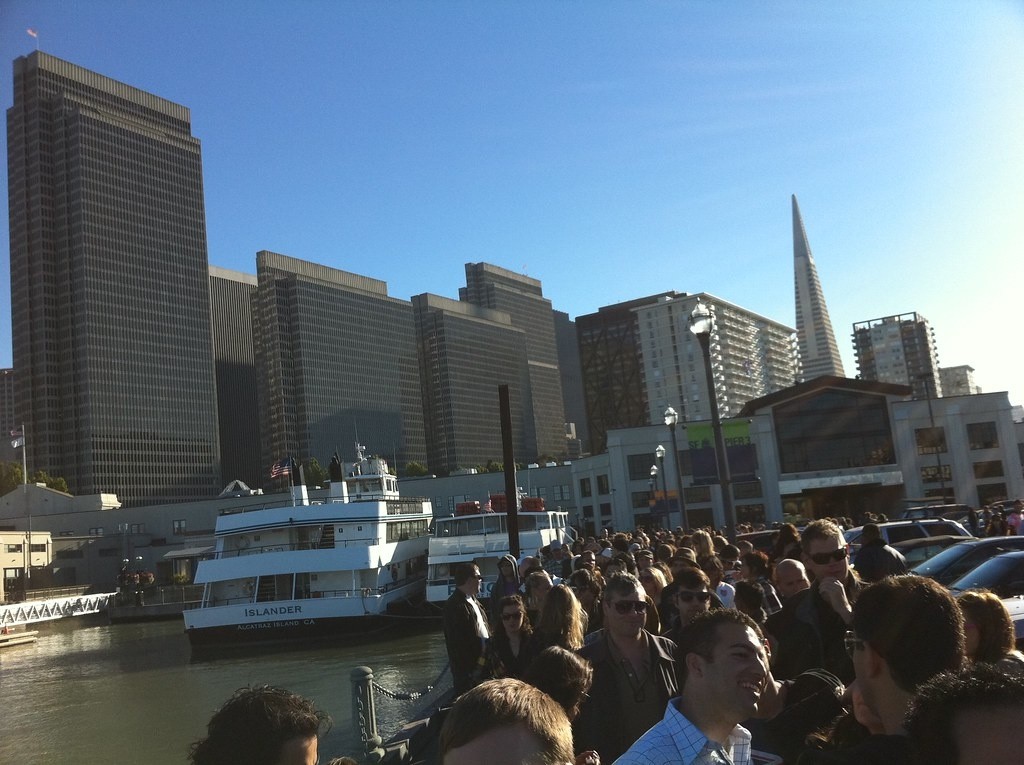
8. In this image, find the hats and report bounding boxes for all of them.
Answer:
[639,550,653,561]
[549,540,562,553]
[667,547,701,571]
[719,544,741,562]
[602,548,613,559]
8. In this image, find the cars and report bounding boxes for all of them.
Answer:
[908,535,1024,655]
[738,530,801,556]
[976,499,1020,518]
[888,531,979,575]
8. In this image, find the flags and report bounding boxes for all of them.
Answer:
[11,437,24,448]
[11,426,23,436]
[270,457,292,478]
[483,500,492,512]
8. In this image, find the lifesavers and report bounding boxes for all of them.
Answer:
[455,501,480,516]
[437,564,449,577]
[391,571,398,579]
[490,494,507,512]
[521,497,546,512]
[235,536,250,549]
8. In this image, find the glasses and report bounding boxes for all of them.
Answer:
[470,575,483,581]
[676,591,711,604]
[804,545,846,565]
[844,631,883,659]
[501,612,523,622]
[608,599,651,615]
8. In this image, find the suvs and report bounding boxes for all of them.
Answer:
[840,517,974,566]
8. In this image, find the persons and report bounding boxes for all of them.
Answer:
[191,498,1024,765]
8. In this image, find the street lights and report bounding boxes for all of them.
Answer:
[918,372,948,503]
[688,296,739,546]
[656,444,673,533]
[649,465,664,529]
[663,404,690,533]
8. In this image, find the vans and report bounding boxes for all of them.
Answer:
[903,504,973,520]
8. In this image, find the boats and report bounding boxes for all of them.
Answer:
[182,420,440,645]
[426,463,578,614]
